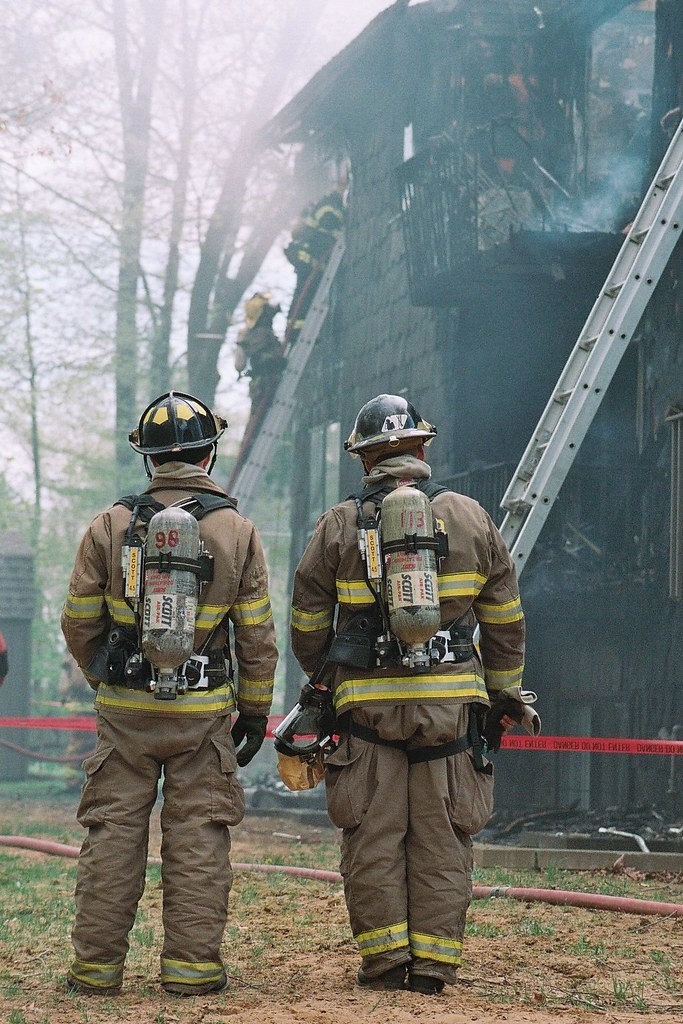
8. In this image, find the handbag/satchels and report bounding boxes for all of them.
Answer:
[483,684,541,755]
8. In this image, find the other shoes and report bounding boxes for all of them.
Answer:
[68,971,120,994]
[356,965,405,989]
[163,972,228,998]
[410,974,444,994]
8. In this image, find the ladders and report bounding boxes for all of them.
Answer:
[230,236,348,519]
[504,115,683,583]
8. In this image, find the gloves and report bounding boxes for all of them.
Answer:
[231,713,267,767]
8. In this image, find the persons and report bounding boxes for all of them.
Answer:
[277,390,526,993]
[283,176,348,342]
[235,291,285,417]
[64,389,277,997]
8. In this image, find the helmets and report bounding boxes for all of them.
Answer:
[129,391,227,455]
[344,394,436,454]
[246,292,269,330]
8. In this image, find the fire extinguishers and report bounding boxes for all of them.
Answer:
[142,505,201,702]
[378,486,445,669]
